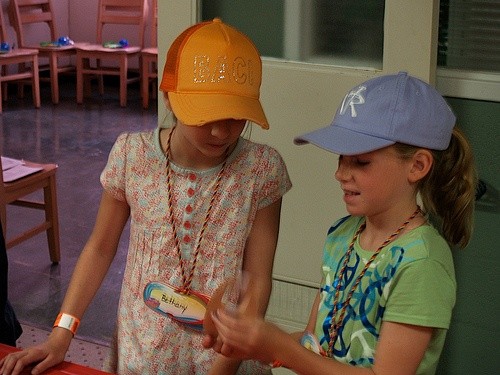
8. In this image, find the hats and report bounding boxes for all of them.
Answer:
[294,72,456,156]
[159,18,269,129]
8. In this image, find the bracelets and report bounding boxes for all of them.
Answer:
[53,313,80,337]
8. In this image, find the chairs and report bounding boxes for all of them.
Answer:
[140,0,159,109]
[72,0,148,108]
[0,2,41,114]
[0,146,63,267]
[10,0,94,104]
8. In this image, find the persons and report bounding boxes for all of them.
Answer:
[200,71,474,375]
[0,18,293,375]
